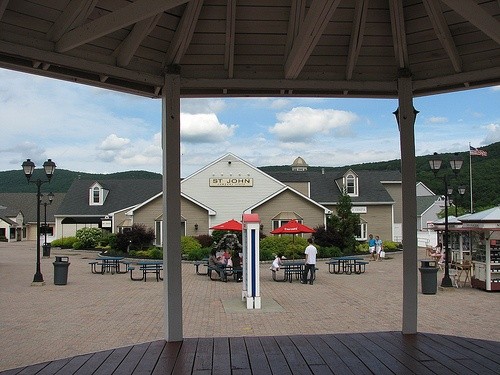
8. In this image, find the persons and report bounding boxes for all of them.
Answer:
[300,238,318,285]
[369,234,376,261]
[376,236,384,261]
[436,243,442,253]
[209,248,226,282]
[270,253,283,271]
[231,252,242,283]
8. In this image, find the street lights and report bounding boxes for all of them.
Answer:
[21,158,57,284]
[448,184,467,268]
[36,191,55,247]
[428,150,463,287]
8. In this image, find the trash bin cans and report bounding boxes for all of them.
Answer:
[43,243,51,256]
[419,260,440,294]
[53,255,70,285]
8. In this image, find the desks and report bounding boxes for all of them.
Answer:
[282,260,306,282]
[135,262,164,282]
[332,257,364,275]
[452,264,472,288]
[95,257,124,275]
[431,253,442,267]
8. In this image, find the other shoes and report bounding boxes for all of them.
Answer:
[300,280,307,284]
[309,280,313,285]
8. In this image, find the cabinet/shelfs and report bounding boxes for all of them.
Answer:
[471,245,500,290]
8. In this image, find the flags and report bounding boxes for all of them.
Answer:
[470,146,487,156]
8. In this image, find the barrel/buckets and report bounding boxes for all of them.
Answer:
[380,250,385,258]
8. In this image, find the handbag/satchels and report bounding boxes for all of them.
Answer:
[228,257,232,267]
[380,250,385,257]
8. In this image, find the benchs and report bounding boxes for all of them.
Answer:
[89,261,164,282]
[194,258,369,282]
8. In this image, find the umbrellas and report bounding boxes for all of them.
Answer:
[210,220,242,231]
[270,220,316,272]
